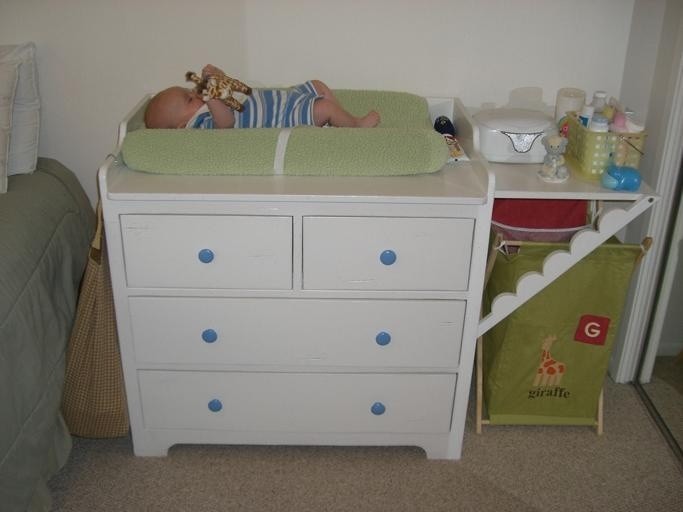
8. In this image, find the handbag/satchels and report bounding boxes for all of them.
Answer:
[59,195,130,440]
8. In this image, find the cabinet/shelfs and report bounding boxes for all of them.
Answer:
[100,93,658,461]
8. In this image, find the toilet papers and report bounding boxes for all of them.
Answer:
[555,87,586,124]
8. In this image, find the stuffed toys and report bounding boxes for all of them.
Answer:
[185,68,252,113]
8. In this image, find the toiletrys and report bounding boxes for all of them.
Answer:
[431,113,469,167]
[608,114,627,166]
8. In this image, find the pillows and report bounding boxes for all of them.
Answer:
[0,43,38,193]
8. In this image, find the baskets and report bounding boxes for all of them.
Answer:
[564,109,650,184]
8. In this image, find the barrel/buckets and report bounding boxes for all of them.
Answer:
[491,199,604,251]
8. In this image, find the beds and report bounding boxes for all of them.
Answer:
[0,158,97,512]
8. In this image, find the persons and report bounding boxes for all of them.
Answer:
[144,63,381,130]
